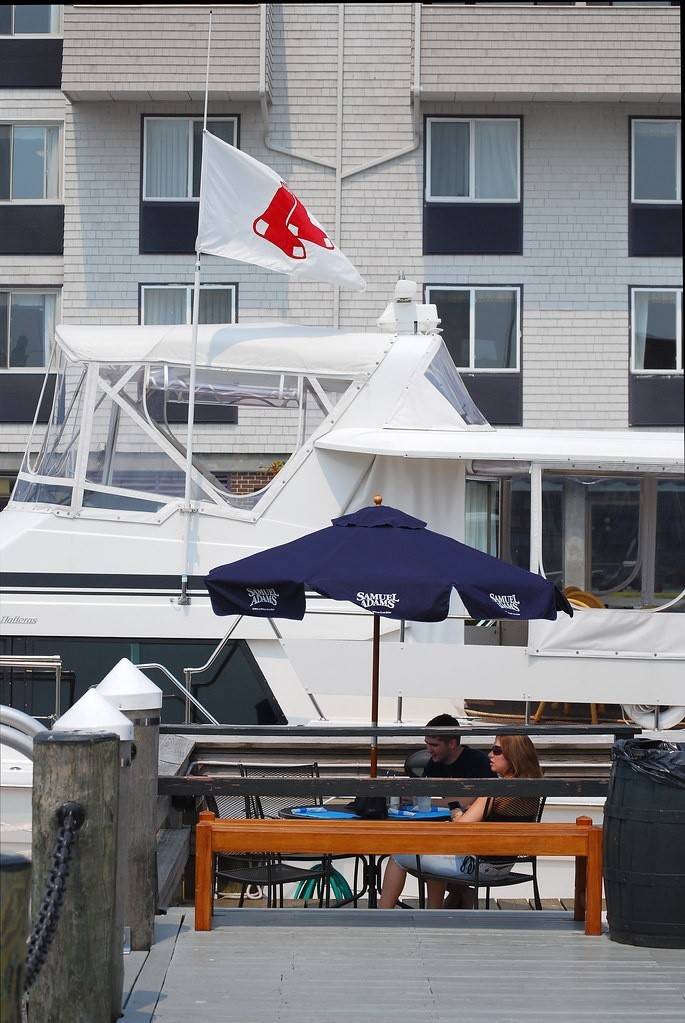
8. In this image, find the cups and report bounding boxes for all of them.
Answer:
[417,797,431,813]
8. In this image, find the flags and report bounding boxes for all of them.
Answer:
[195,128,368,294]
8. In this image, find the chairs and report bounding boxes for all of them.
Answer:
[213,762,546,911]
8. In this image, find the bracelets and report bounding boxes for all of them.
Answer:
[452,813,463,822]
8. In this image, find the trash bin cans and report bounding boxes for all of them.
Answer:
[600,738,684,950]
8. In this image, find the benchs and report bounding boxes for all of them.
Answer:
[195,809,603,935]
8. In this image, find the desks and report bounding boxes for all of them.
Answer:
[279,804,452,908]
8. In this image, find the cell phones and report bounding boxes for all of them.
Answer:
[448,801,464,812]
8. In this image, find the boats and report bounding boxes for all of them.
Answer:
[0,274,684,874]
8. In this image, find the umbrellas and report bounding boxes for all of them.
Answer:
[203,494,573,778]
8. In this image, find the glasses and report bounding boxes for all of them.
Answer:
[492,746,502,755]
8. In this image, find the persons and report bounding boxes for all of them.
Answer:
[376,735,545,909]
[400,712,498,910]
